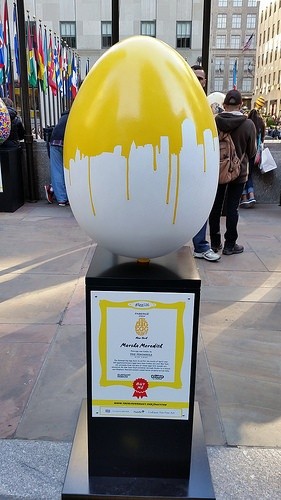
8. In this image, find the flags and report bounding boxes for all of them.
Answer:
[0,0,19,113]
[60,46,82,100]
[242,33,253,52]
[26,11,62,97]
[232,60,238,89]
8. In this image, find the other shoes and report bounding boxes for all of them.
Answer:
[239,200,249,207]
[249,198,257,208]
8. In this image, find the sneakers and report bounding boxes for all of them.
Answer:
[44,185,53,204]
[222,244,244,256]
[211,243,222,252]
[191,248,220,261]
[57,202,65,207]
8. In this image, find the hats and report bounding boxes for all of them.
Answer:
[224,89,242,105]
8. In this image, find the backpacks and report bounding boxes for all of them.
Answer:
[217,128,245,184]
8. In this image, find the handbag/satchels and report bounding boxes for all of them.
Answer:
[256,143,277,174]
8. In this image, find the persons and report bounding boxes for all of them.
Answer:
[44,107,70,206]
[188,65,281,262]
[0,97,27,202]
